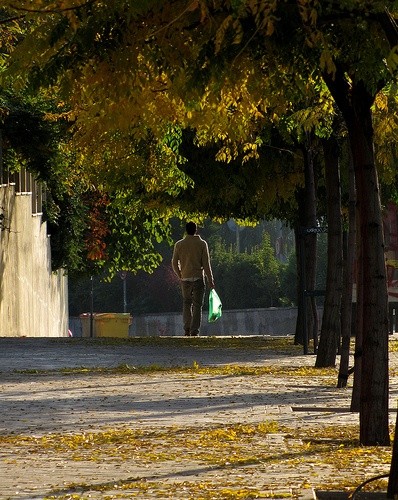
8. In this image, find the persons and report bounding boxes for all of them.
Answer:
[171,221,215,336]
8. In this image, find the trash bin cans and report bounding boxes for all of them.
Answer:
[93,313,133,337]
[80,312,96,338]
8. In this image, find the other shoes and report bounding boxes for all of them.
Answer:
[191,333,199,336]
[185,332,190,336]
